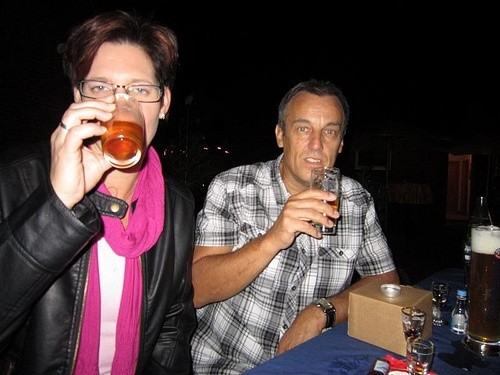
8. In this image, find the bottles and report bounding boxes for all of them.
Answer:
[450,290,470,341]
[463,237,471,270]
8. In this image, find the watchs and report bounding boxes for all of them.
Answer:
[311,298,336,333]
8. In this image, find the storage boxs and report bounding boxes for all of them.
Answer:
[347,281,433,358]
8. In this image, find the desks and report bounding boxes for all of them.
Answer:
[239,265,500,375]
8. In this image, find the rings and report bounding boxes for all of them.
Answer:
[58,121,70,131]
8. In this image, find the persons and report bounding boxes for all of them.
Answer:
[0,9,197,375]
[191,78,399,375]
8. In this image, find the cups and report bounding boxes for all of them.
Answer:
[311,168,343,235]
[401,308,426,358]
[431,280,448,326]
[406,337,434,375]
[100,94,145,169]
[462,224,500,356]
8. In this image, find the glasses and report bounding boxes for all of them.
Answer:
[76,79,164,104]
